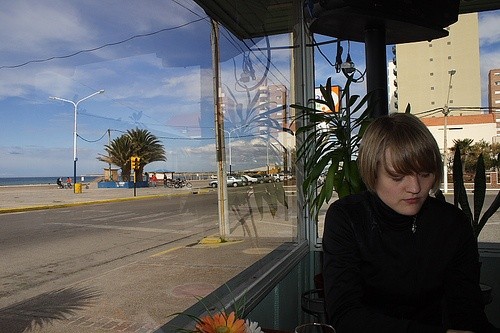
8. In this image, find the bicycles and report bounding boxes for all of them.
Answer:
[56,183,72,189]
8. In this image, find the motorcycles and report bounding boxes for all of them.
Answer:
[166,178,192,188]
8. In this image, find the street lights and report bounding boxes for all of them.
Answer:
[48,89,104,194]
[258,137,276,174]
[212,124,249,176]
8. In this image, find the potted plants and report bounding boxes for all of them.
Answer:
[434,141,500,306]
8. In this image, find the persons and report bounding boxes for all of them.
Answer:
[57,177,71,188]
[322,113,497,333]
[144,172,168,187]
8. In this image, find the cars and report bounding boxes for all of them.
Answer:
[209,174,258,189]
[251,173,292,183]
